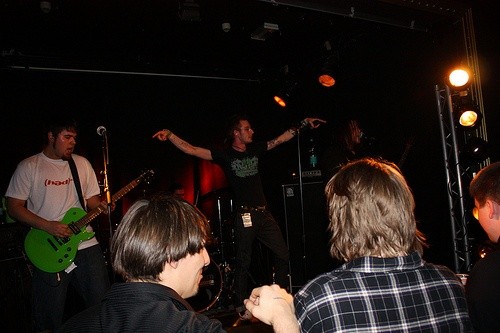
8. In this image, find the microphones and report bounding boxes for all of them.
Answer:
[96,126,107,136]
[295,120,307,130]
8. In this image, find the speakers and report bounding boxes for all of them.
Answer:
[280,180,343,291]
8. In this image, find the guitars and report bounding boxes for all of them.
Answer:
[24,169,154,273]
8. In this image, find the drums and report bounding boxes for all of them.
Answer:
[205,232,221,256]
[182,257,231,313]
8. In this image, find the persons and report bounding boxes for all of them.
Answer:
[152,114,327,308]
[293,156,474,333]
[335,117,366,171]
[56,191,228,333]
[4,112,116,333]
[469,161,500,333]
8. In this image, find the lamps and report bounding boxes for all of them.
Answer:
[448,68,473,93]
[467,137,494,160]
[317,54,340,89]
[273,81,301,109]
[459,105,484,129]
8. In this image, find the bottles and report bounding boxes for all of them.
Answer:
[305,138,321,171]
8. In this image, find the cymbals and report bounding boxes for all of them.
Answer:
[199,186,235,202]
